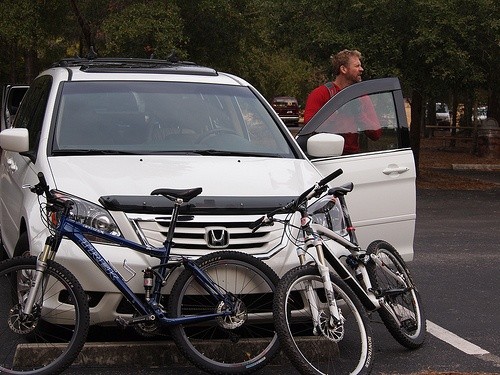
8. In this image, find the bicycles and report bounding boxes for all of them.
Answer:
[249,168,428,375]
[0,171,291,375]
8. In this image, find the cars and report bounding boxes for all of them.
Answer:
[472,107,488,126]
[1,85,31,132]
[0,59,416,344]
[425,102,451,131]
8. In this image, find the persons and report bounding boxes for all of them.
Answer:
[302,48,383,156]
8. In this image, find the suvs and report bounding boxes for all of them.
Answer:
[271,96,300,127]
